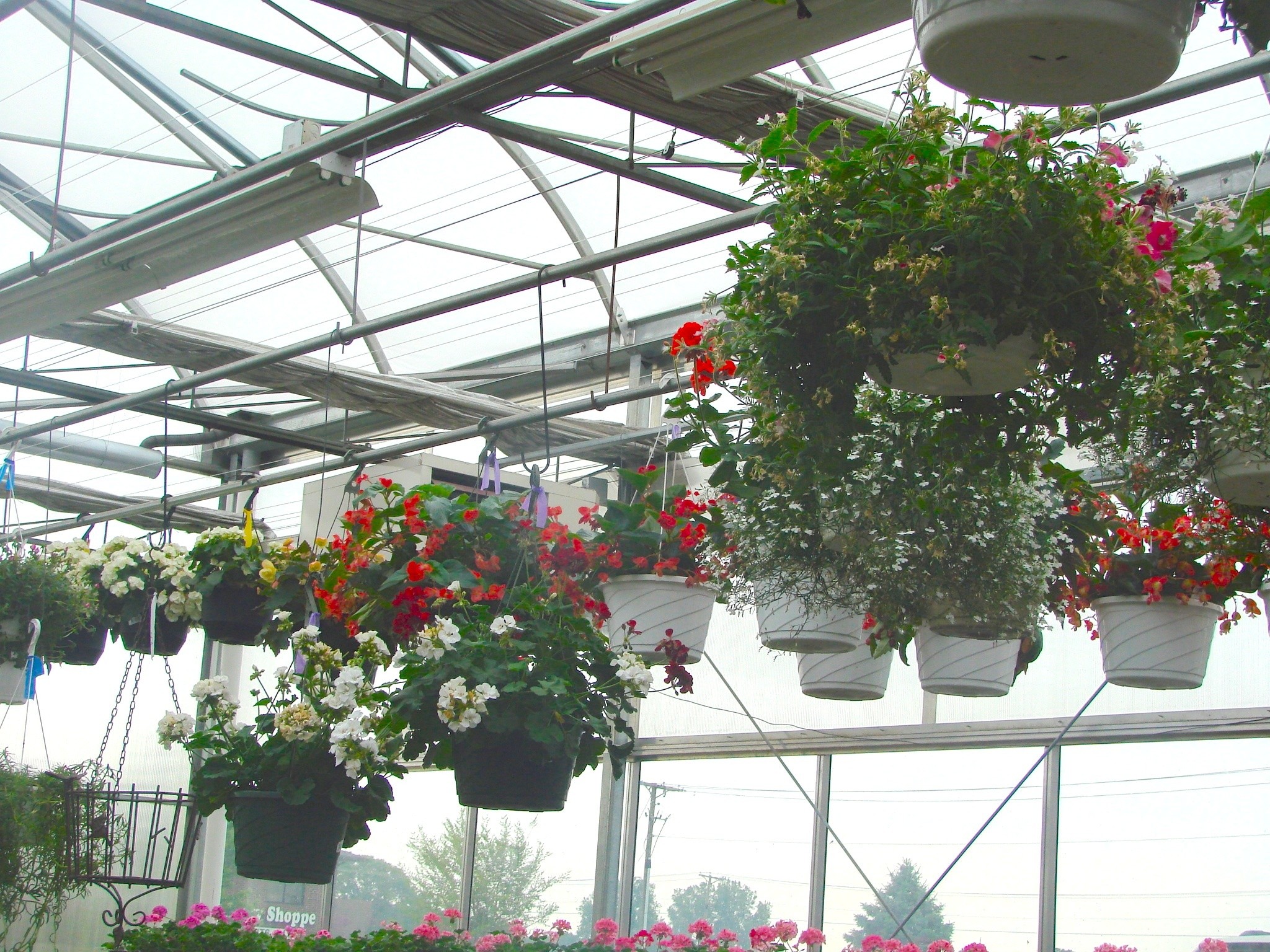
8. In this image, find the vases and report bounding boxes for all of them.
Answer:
[600,570,718,665]
[44,594,105,667]
[202,573,274,647]
[226,789,345,885]
[747,555,864,653]
[928,552,1030,640]
[1199,348,1270,508]
[121,582,189,657]
[1092,597,1222,692]
[796,589,894,701]
[866,319,1046,396]
[446,695,577,812]
[914,605,1025,697]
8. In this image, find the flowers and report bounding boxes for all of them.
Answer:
[0,0,1270,887]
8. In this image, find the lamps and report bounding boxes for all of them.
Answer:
[0,152,379,356]
[575,1,921,101]
